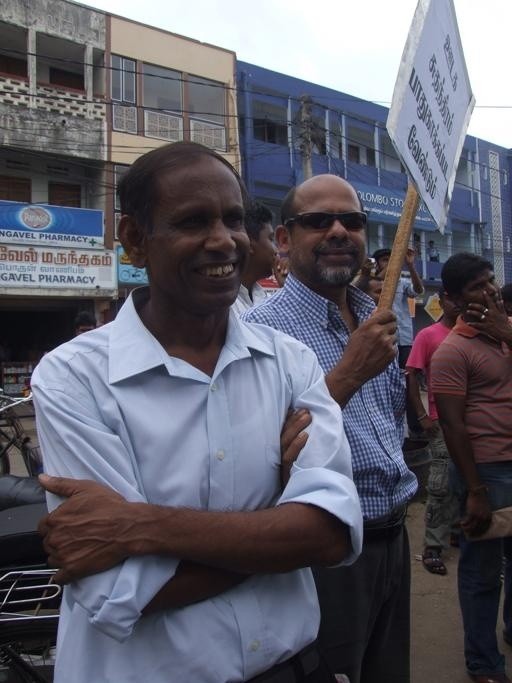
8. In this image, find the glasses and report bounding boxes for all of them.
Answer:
[282,209,371,233]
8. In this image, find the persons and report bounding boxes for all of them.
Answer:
[428,250,511,683]
[370,243,428,439]
[400,286,464,575]
[229,196,289,318]
[237,172,420,682]
[27,139,368,681]
[351,252,379,294]
[72,309,97,336]
[497,282,512,325]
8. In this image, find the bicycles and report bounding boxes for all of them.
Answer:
[0,386,44,480]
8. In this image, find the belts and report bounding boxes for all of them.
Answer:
[363,502,413,543]
[246,639,321,683]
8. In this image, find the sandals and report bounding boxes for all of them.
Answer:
[423,551,447,576]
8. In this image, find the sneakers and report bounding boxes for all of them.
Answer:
[469,673,512,683]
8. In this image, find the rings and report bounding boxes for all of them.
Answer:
[483,307,489,313]
[480,314,487,320]
[497,299,505,305]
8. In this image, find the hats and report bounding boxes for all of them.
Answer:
[371,248,391,258]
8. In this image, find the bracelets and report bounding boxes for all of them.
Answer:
[467,483,487,491]
[417,412,428,421]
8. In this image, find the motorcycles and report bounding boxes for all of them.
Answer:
[0,471,66,683]
